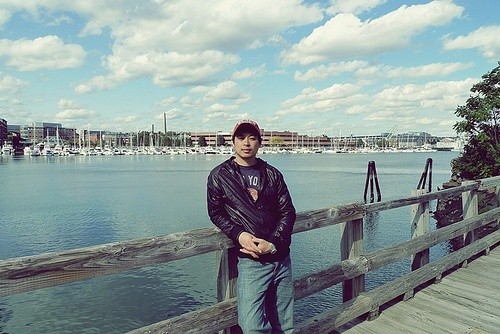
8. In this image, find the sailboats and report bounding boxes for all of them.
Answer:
[0,121,455,156]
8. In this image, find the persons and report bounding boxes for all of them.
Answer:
[207,118,297,334]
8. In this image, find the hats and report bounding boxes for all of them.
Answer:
[232,119,260,139]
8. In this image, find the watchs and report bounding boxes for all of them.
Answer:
[271,243,277,256]
